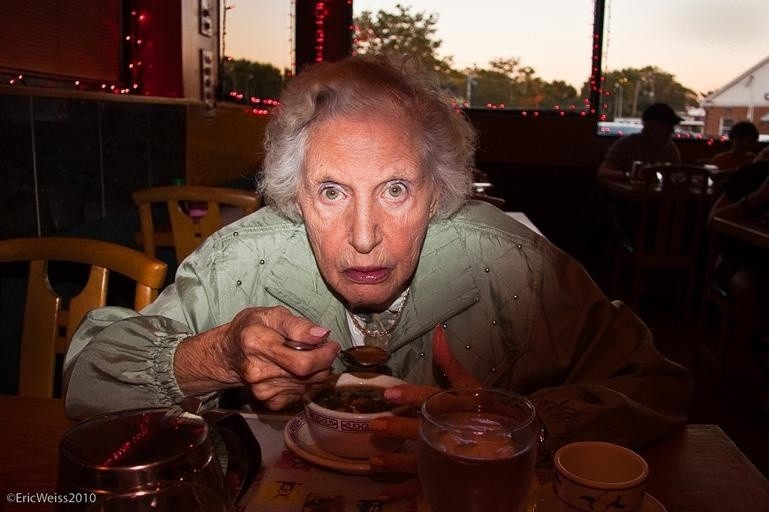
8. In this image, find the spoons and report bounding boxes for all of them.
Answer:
[282,337,392,369]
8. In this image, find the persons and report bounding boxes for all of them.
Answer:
[61,45,694,503]
[705,150,769,369]
[347,293,409,339]
[710,120,761,170]
[597,101,687,310]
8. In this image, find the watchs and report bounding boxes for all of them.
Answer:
[537,423,556,464]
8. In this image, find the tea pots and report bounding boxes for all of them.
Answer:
[51,394,263,511]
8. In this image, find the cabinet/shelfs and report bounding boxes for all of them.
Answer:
[132,185,263,261]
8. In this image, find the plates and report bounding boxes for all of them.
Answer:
[524,479,667,512]
[282,407,412,476]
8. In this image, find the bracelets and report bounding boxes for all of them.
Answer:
[738,196,754,214]
[623,170,632,183]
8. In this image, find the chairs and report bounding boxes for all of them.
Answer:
[610,165,709,313]
[0,237,168,399]
[699,169,738,361]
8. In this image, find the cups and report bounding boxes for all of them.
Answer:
[416,384,540,512]
[550,439,650,512]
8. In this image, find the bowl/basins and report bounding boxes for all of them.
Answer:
[303,372,414,461]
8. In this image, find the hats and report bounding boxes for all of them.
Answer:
[642,102,685,123]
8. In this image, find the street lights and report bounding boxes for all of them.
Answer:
[617,83,633,118]
[466,73,482,109]
